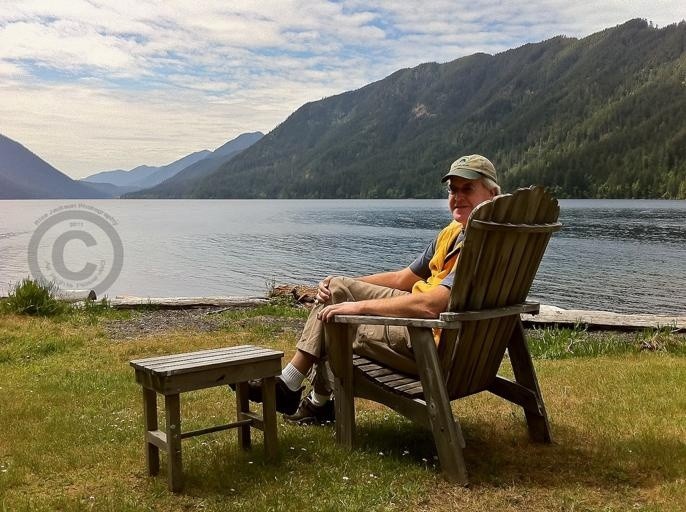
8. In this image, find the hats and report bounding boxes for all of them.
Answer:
[441,154,497,183]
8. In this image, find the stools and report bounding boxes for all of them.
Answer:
[129,345,285,493]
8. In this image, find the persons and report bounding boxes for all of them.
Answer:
[227,151,502,427]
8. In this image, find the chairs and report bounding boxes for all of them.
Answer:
[314,183,566,488]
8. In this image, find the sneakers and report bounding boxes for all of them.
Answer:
[282,389,337,426]
[228,376,306,415]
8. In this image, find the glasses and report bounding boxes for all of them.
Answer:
[447,183,475,196]
